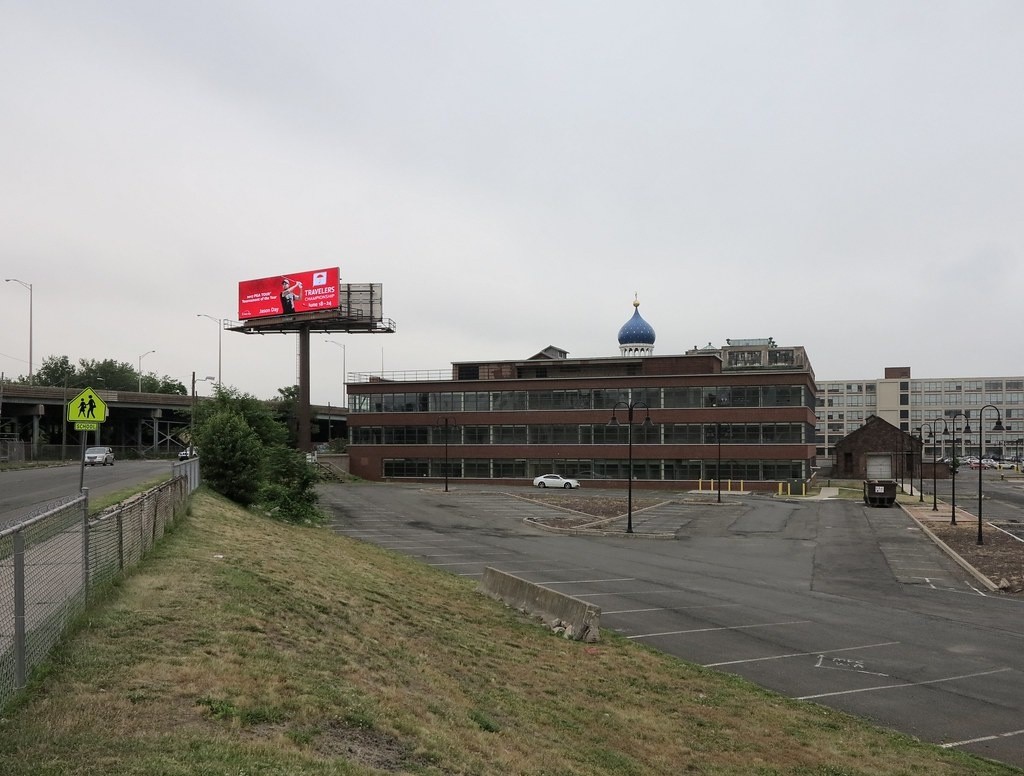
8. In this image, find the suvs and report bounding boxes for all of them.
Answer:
[84,447,115,466]
[178,447,198,461]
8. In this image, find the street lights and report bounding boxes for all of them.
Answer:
[139,351,155,393]
[932,418,950,511]
[895,424,934,502]
[325,340,345,408]
[189,372,215,459]
[950,413,973,525]
[432,416,459,492]
[197,314,221,390]
[975,405,1006,545]
[705,421,734,502]
[605,401,655,534]
[6,279,33,385]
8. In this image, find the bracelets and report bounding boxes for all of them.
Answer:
[299,286,302,289]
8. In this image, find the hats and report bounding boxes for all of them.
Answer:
[282,280,289,284]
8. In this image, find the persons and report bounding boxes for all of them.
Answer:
[938,456,960,469]
[279,279,302,314]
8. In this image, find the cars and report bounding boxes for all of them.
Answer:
[936,453,1023,470]
[533,474,580,489]
[577,471,611,479]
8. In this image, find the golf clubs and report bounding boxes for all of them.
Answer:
[280,276,296,283]
